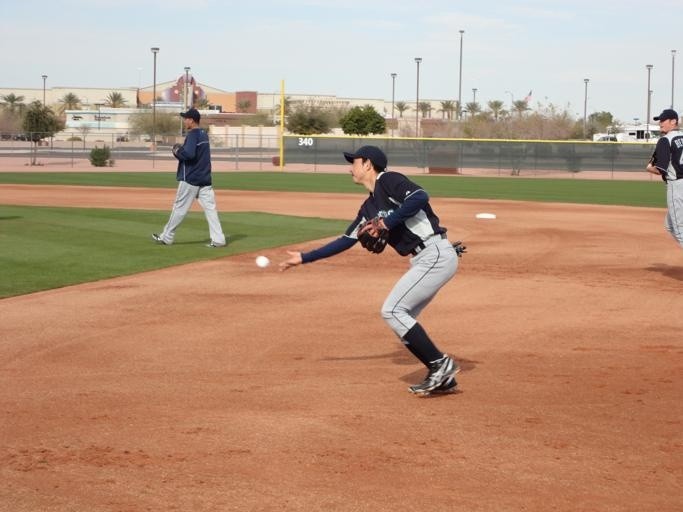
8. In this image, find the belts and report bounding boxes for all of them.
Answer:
[409,232,447,257]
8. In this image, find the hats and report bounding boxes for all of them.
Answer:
[180,109,201,121]
[653,109,678,122]
[343,145,387,171]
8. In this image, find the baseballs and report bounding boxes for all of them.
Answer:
[256,256,269,268]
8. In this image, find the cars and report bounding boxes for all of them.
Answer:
[12,131,41,142]
[0,130,11,140]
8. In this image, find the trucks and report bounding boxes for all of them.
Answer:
[593,124,660,142]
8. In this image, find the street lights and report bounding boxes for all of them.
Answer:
[645,64,652,123]
[41,75,48,106]
[669,49,676,111]
[414,56,423,138]
[471,88,477,102]
[580,78,589,139]
[389,72,397,136]
[457,29,465,107]
[183,65,190,113]
[150,45,160,143]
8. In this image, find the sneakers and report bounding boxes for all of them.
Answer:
[151,233,165,245]
[410,354,461,395]
[416,378,459,398]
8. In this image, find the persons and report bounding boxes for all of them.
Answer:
[150,108,226,248]
[279,145,462,398]
[647,109,683,246]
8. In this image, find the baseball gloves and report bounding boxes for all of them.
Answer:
[357,216,390,254]
